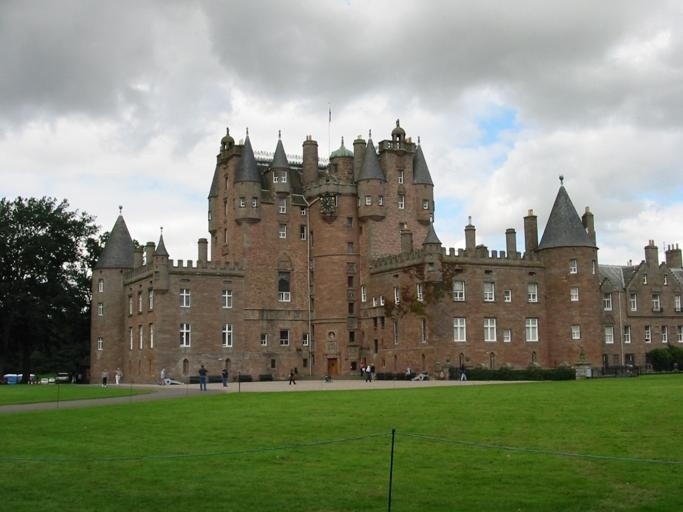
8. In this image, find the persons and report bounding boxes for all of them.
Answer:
[288,367,296,385]
[198,364,208,391]
[114,367,122,384]
[221,367,227,387]
[160,368,165,384]
[359,361,376,384]
[100,367,109,388]
[458,364,467,381]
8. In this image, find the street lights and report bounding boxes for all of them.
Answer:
[276,188,341,381]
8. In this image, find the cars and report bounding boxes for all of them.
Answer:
[41,377,55,384]
[56,373,72,384]
